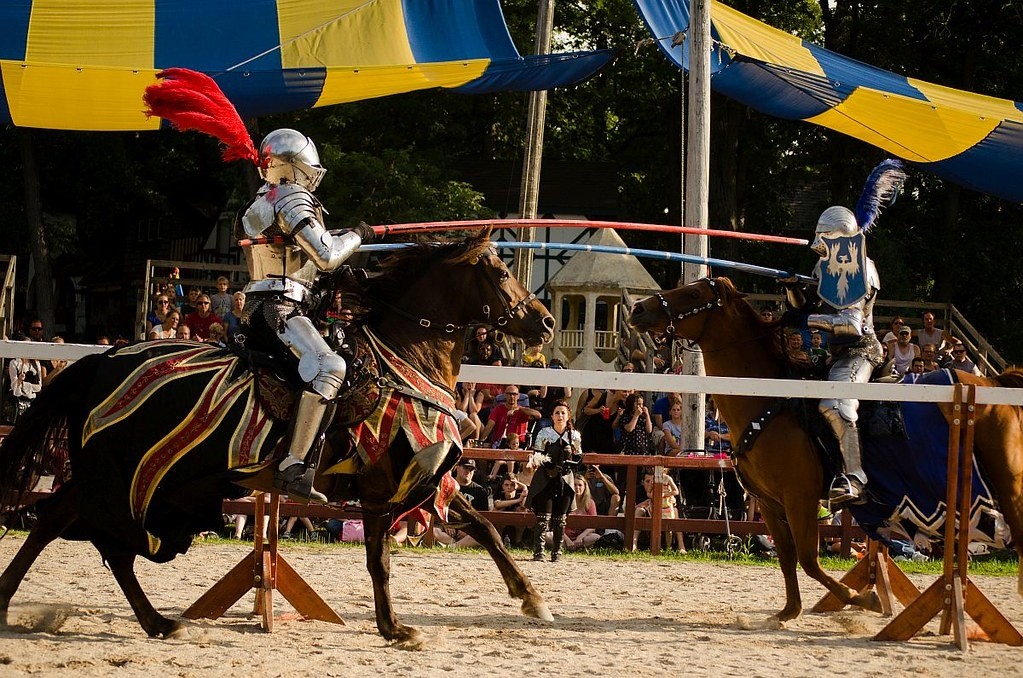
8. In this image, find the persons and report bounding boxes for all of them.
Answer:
[234,128,374,504]
[251,323,772,552]
[760,304,774,323]
[339,306,354,326]
[871,342,889,382]
[807,332,832,367]
[942,345,981,377]
[3,266,253,541]
[796,506,992,564]
[787,331,810,364]
[911,356,926,374]
[920,342,940,372]
[778,207,884,506]
[916,312,961,356]
[888,325,921,375]
[882,315,906,346]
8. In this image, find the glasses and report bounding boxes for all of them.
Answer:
[505,392,518,396]
[156,300,169,305]
[475,332,487,337]
[480,347,491,351]
[883,348,887,352]
[30,327,44,331]
[953,350,966,354]
[760,313,772,317]
[197,301,209,305]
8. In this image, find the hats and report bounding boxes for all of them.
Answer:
[459,458,477,471]
[898,325,912,335]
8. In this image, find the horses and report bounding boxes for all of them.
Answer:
[628,274,1023,626]
[0,224,558,654]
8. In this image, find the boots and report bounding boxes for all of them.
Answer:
[551,514,567,562]
[533,515,551,563]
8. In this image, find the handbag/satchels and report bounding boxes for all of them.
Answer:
[340,519,365,543]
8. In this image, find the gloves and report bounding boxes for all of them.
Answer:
[776,267,798,289]
[780,310,808,330]
[353,220,374,243]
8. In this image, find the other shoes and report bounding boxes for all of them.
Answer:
[308,531,317,542]
[281,531,291,539]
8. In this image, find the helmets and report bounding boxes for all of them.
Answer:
[255,129,326,193]
[810,206,857,258]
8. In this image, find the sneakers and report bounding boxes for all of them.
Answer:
[272,458,329,505]
[829,474,868,506]
[894,551,929,564]
